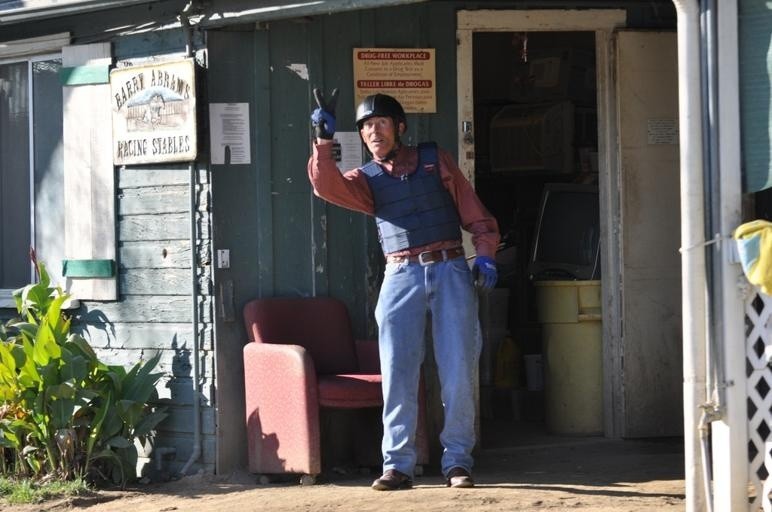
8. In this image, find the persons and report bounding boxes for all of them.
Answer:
[307,88,501,491]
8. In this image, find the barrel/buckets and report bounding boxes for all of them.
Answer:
[523,354,543,391]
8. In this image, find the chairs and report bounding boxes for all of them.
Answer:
[241,298,430,487]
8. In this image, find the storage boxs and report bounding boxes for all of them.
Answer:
[487,99,574,172]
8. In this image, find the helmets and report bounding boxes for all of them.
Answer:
[354,93,407,130]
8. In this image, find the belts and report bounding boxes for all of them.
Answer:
[386,244,465,266]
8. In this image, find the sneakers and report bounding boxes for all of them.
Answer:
[371,470,413,490]
[446,467,474,488]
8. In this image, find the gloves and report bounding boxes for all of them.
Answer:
[470,255,498,297]
[310,86,340,140]
